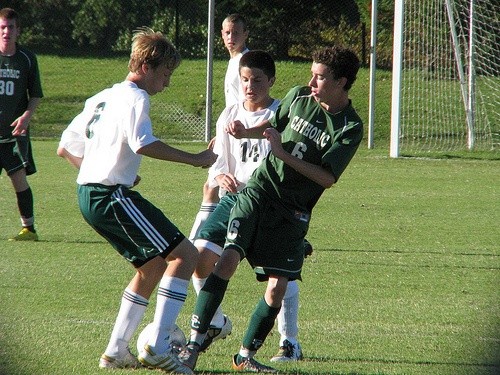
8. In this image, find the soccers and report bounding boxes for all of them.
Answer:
[137,322,186,364]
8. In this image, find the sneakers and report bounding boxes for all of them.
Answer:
[270,338,304,363]
[137,344,194,375]
[8,228,38,242]
[233,353,277,374]
[199,314,233,353]
[98,349,146,370]
[177,340,200,372]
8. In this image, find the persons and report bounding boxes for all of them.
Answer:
[56,28,197,374]
[177,44,365,373]
[192,51,306,363]
[0,7,44,242]
[188,15,249,228]
